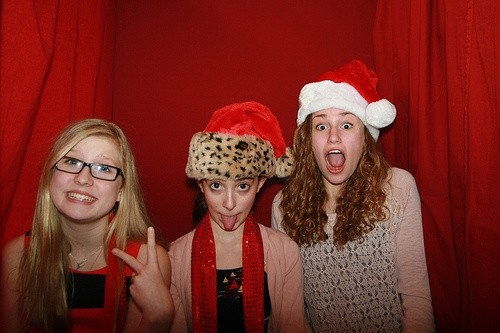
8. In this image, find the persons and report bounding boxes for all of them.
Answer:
[167,101,312,333]
[0,118,174,333]
[270,60,434,333]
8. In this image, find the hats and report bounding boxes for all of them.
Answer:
[296,60,397,143]
[185,101,296,182]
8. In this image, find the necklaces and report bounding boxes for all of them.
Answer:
[68,246,104,269]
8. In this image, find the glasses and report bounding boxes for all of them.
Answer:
[55,155,126,183]
